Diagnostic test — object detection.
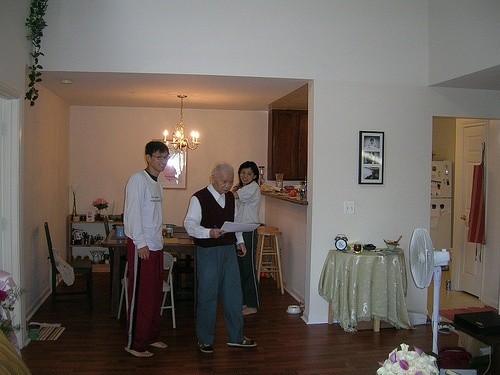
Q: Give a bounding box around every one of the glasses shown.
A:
[150,155,170,160]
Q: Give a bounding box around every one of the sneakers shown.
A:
[198,342,213,353]
[226,336,257,347]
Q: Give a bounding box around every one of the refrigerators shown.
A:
[430,161,451,270]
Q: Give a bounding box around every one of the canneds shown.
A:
[166,225,173,238]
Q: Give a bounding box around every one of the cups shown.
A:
[276,174,283,193]
[446,280,451,290]
[354,244,361,251]
[116,226,124,240]
[165,228,173,238]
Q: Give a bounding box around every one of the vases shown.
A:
[97,209,101,222]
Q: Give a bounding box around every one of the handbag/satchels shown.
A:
[52,248,74,286]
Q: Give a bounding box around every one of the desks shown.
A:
[102,227,197,316]
[318,248,416,332]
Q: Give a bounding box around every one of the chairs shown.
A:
[44,222,93,316]
[118,251,176,328]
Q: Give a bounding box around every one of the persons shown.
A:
[230,160,261,315]
[183,164,257,353]
[368,138,376,149]
[122,141,171,358]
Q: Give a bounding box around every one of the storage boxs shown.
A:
[453,311,500,349]
[458,330,491,358]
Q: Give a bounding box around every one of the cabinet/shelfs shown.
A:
[267,109,308,181]
[67,214,125,273]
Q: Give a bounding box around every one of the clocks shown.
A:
[335,233,349,252]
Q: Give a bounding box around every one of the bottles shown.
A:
[259,166,265,186]
[300,181,305,197]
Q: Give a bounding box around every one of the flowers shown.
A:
[376,343,440,375]
[91,197,109,210]
[0,288,26,338]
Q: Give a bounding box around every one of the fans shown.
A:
[409,227,450,355]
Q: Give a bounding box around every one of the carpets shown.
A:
[439,305,499,322]
[27,322,65,341]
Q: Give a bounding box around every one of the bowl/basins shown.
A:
[286,305,301,314]
[386,244,397,249]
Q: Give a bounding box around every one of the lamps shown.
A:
[162,94,200,151]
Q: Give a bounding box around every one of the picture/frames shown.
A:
[153,138,187,189]
[359,131,384,185]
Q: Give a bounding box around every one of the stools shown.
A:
[256,225,284,295]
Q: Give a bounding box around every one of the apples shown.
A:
[290,190,297,197]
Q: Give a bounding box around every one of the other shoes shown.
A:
[242,305,257,315]
[124,347,154,357]
[148,342,168,348]
